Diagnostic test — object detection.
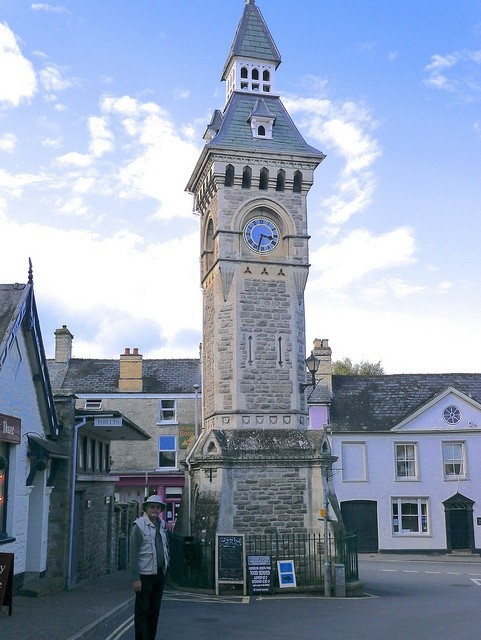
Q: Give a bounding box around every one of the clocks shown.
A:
[242,215,282,257]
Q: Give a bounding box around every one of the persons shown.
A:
[130,495,170,639]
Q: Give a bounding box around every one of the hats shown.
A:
[143,495,166,512]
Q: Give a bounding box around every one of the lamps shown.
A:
[302,350,322,391]
[22,431,47,471]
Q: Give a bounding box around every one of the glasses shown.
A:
[146,505,162,509]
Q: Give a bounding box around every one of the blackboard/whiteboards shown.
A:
[215,533,247,584]
[248,555,276,595]
